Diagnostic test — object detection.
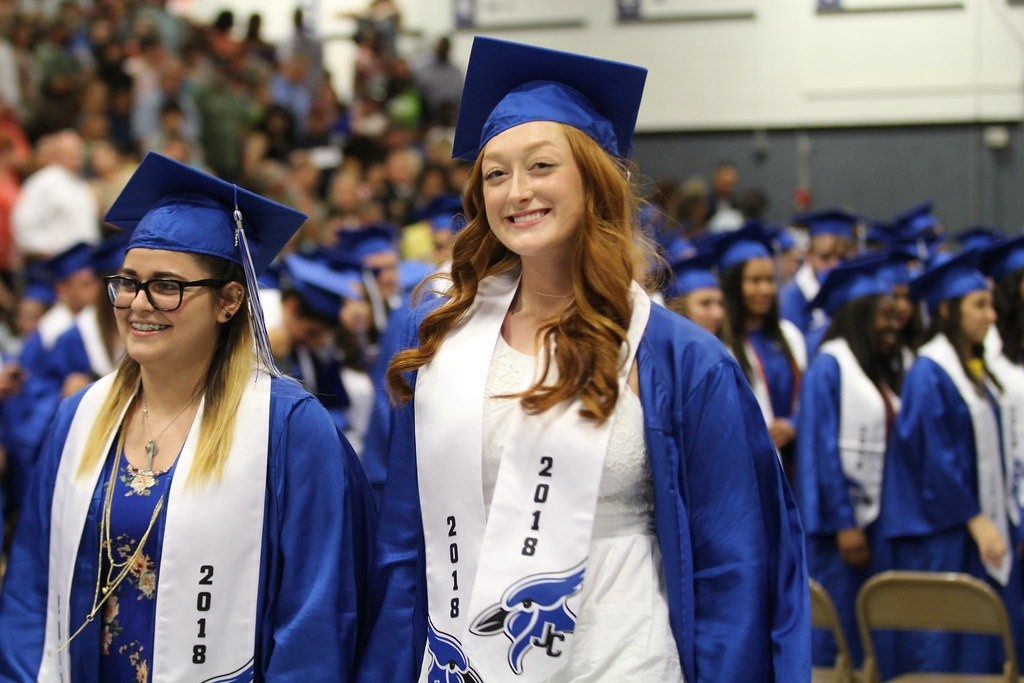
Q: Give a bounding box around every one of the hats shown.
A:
[104,151,309,383]
[649,219,793,301]
[797,198,1024,315]
[44,242,100,279]
[429,194,470,231]
[451,35,647,175]
[287,220,401,319]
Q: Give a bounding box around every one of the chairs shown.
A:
[807,575,854,683]
[853,569,1024,683]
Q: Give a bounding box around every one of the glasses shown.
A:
[105,274,220,312]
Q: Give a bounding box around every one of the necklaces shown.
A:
[139,392,203,471]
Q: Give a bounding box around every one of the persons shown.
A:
[0,152,356,683]
[795,258,915,672]
[886,247,1021,675]
[0,1,1024,505]
[350,36,811,683]
